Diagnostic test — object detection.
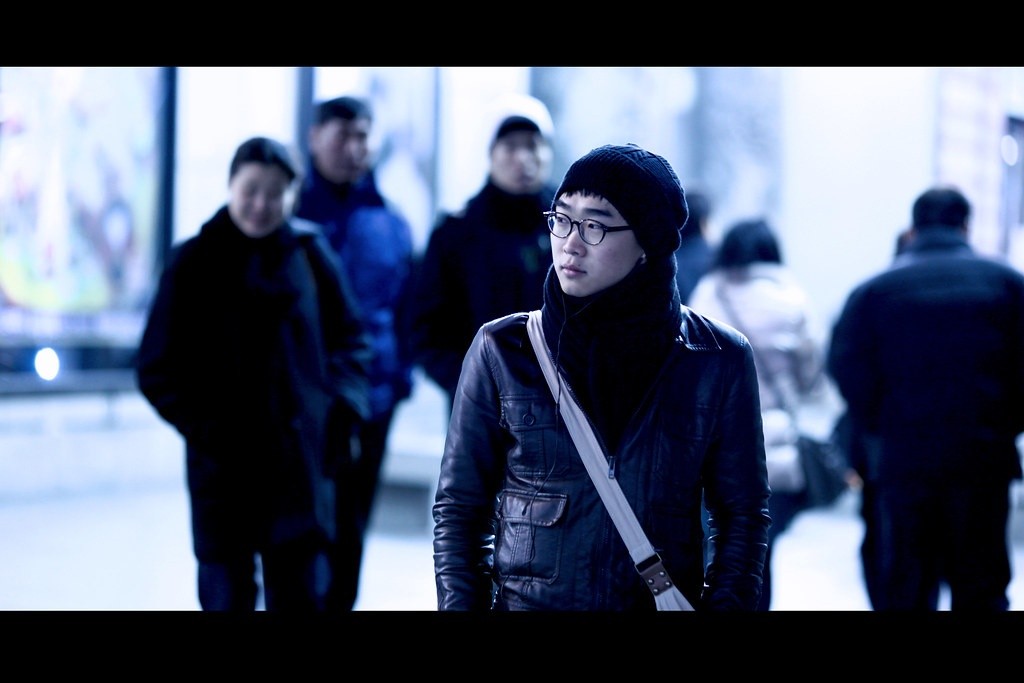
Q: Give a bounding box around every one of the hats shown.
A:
[550,142,689,269]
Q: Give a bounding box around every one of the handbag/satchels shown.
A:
[797,436,848,507]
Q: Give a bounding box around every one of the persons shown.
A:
[135,138,368,611]
[823,187,1023,613]
[432,144,771,612]
[676,194,717,305]
[290,98,415,611]
[685,221,848,541]
[397,96,563,419]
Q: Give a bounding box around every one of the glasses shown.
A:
[543,211,631,246]
